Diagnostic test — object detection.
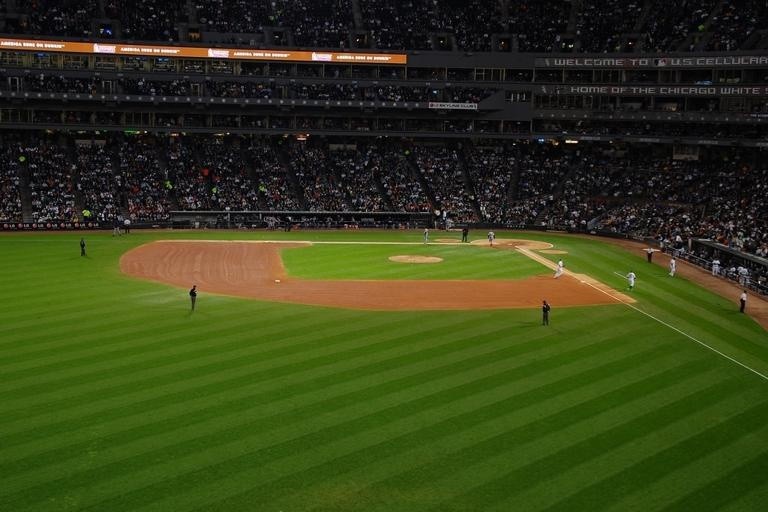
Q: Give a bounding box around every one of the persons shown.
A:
[541,299,551,326]
[423,226,429,246]
[461,226,468,242]
[538,1,767,302]
[284,214,293,232]
[3,0,536,230]
[189,285,198,311]
[739,289,748,313]
[79,237,86,256]
[112,217,122,236]
[124,217,131,235]
[487,229,495,247]
